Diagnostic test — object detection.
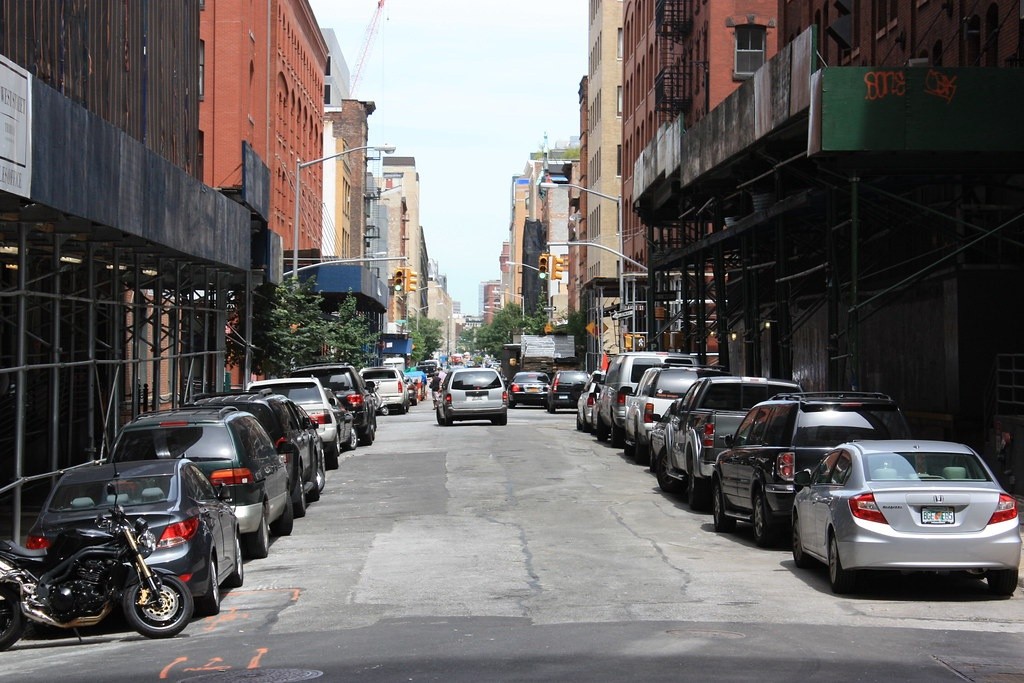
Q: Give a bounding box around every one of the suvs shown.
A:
[290,363,377,445]
[590,353,700,449]
[712,391,927,548]
[106,375,340,559]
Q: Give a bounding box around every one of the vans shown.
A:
[434,368,509,427]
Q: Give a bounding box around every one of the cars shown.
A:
[357,355,427,418]
[507,371,590,414]
[324,388,358,450]
[27,458,244,615]
[792,440,1022,596]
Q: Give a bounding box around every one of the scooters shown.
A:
[373,380,389,416]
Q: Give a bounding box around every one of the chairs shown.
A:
[141,487,165,502]
[875,468,899,479]
[106,494,128,504]
[70,498,95,509]
[942,466,966,480]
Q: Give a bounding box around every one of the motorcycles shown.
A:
[0,483,194,652]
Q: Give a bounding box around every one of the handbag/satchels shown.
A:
[429,383,433,388]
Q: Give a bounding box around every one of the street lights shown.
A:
[541,182,625,353]
[406,285,443,332]
[547,243,676,352]
[416,302,443,333]
[492,291,525,335]
[291,144,396,369]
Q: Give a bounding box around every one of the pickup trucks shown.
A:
[620,364,807,511]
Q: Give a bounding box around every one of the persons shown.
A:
[438,367,447,381]
[429,371,444,410]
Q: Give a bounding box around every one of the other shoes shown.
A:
[433,409,435,410]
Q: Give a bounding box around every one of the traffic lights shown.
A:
[404,267,417,294]
[393,269,404,296]
[551,255,564,280]
[539,255,548,280]
[623,333,645,349]
